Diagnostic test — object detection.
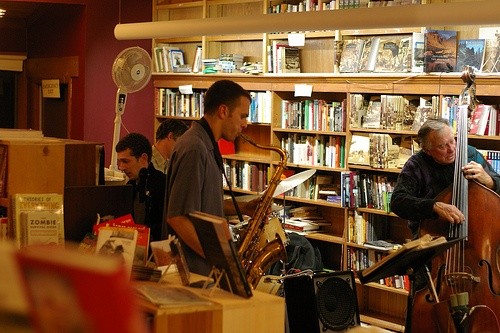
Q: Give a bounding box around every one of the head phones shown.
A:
[139,167,157,179]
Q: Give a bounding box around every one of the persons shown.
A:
[115,80,252,275]
[390,115,500,333]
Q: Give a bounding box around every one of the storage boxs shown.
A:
[13,239,138,333]
[130,282,224,333]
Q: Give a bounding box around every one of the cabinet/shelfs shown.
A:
[152,0,500,333]
[0,136,104,244]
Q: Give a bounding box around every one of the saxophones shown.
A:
[238,132,289,292]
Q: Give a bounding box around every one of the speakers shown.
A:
[282,270,360,333]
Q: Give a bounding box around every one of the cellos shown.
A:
[404,69,500,333]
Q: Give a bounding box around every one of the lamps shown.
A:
[112,0,500,40]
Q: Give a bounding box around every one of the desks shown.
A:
[162,271,286,333]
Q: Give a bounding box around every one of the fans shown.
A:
[103,45,153,182]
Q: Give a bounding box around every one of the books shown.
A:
[362,234,447,277]
[8,192,65,249]
[350,94,440,129]
[334,27,500,73]
[275,170,396,213]
[347,134,421,168]
[204,40,302,73]
[156,88,346,131]
[441,97,497,136]
[477,150,500,174]
[223,159,269,192]
[217,138,235,156]
[275,203,332,235]
[280,132,344,168]
[346,209,410,290]
[154,45,202,73]
[268,0,412,13]
[77,213,253,307]
